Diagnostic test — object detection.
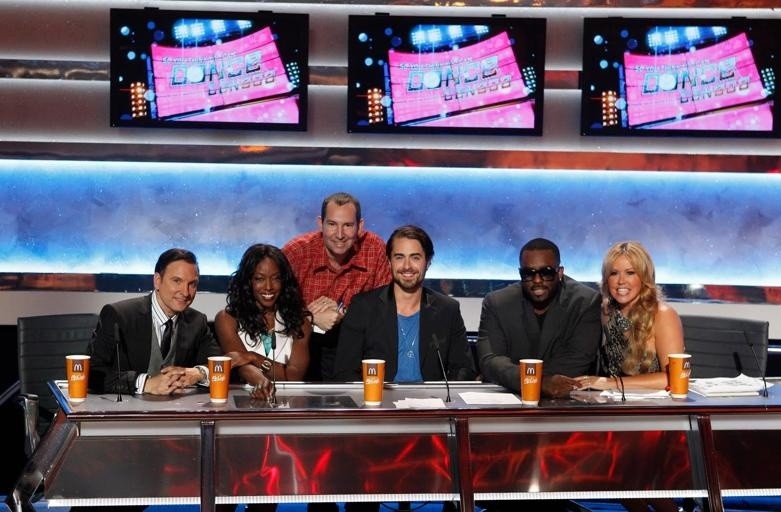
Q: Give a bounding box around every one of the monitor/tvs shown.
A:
[108,6,311,132]
[580,16,777,140]
[346,12,547,138]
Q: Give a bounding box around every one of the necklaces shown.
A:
[392,285,423,361]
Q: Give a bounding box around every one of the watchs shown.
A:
[195,367,206,383]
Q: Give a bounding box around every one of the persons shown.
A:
[477,237,602,397]
[573,241,683,391]
[214,244,312,401]
[280,192,393,333]
[334,226,476,382]
[84,248,223,396]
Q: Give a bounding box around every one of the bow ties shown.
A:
[161,320,173,360]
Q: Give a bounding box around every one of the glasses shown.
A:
[518,265,559,281]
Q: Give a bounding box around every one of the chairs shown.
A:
[679,313,769,381]
[16,312,99,432]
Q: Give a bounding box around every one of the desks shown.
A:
[49,375,781,511]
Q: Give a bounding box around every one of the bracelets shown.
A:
[260,357,273,374]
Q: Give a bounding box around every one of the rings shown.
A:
[323,302,328,305]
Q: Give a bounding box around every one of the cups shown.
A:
[668,353,692,400]
[208,356,231,403]
[362,359,385,406]
[519,359,543,406]
[65,355,91,402]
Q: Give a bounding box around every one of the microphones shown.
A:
[430,332,452,403]
[603,322,626,402]
[112,321,124,403]
[270,328,278,407]
[742,328,769,399]
